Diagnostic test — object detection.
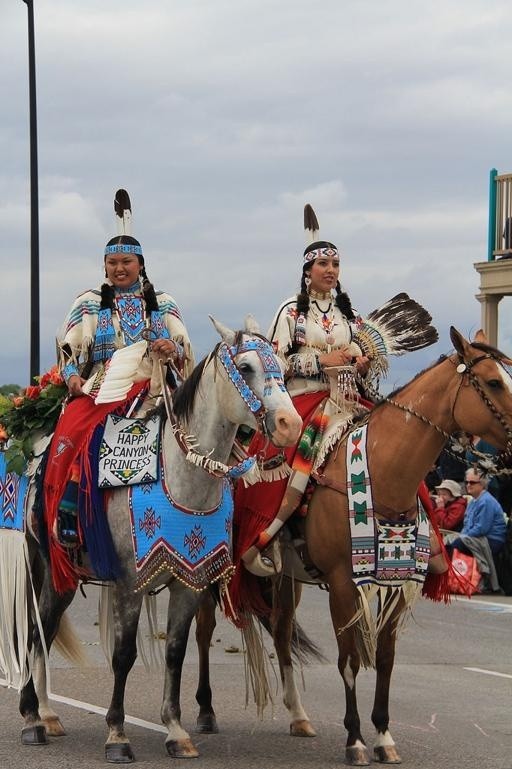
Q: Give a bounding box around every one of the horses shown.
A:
[194,323,512,768]
[0,314,305,769]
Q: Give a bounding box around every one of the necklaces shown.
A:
[309,289,337,324]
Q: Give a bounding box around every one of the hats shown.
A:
[434,478,464,498]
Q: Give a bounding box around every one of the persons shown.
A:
[424,431,511,594]
[35,235,195,551]
[242,241,449,576]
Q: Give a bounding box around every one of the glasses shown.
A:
[462,479,482,486]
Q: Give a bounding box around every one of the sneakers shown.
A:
[51,507,81,549]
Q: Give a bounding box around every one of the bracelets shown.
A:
[61,364,79,386]
[171,340,184,358]
[315,354,322,371]
[358,369,371,382]
